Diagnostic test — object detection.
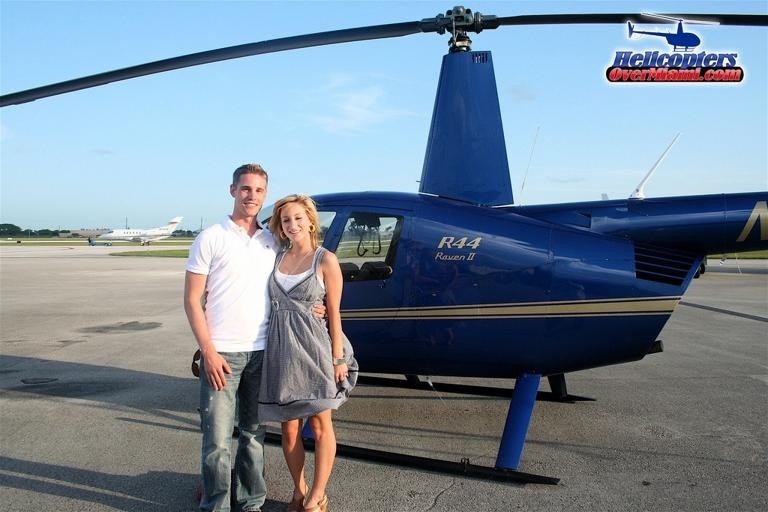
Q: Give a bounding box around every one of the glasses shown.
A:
[191,349,201,377]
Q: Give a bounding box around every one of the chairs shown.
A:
[338,261,393,282]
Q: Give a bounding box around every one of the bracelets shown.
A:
[332,358,347,365]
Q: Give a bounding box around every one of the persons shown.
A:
[257,192,361,512]
[182,161,330,512]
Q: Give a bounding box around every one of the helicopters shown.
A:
[0,6,768,471]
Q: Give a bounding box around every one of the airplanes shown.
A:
[99,215,185,246]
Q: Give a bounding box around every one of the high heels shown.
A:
[287,487,328,512]
